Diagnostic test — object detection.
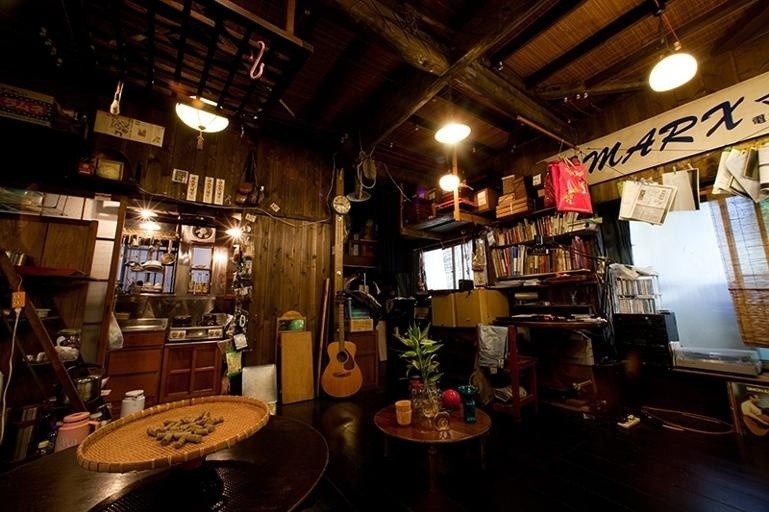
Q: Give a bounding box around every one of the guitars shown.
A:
[321,292,363,399]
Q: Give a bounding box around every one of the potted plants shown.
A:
[394,322,445,430]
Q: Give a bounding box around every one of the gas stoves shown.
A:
[168,324,224,343]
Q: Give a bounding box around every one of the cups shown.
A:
[396,400,411,426]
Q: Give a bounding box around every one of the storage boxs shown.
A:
[455,290,510,328]
[431,293,456,328]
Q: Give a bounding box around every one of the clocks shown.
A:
[332,195,351,215]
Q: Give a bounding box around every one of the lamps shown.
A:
[175,99,230,133]
[648,7,698,92]
[439,166,460,193]
[434,84,471,146]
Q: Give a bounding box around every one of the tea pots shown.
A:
[67,362,104,405]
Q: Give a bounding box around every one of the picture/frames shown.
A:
[95,159,125,180]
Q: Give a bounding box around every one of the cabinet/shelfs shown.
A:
[398,182,555,243]
[486,217,608,420]
[103,330,165,421]
[344,233,381,276]
[612,310,680,407]
[345,331,379,392]
[158,344,222,402]
[0,214,98,329]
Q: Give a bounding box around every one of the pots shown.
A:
[161,240,176,265]
[197,314,217,325]
[173,313,191,325]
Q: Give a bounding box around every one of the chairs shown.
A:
[477,324,538,424]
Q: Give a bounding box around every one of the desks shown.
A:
[672,365,769,434]
[375,403,492,512]
[1,415,330,512]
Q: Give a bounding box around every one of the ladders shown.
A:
[0,246,113,428]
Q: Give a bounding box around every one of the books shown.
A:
[491,238,593,278]
[495,211,597,246]
[616,276,660,314]
[496,197,533,218]
